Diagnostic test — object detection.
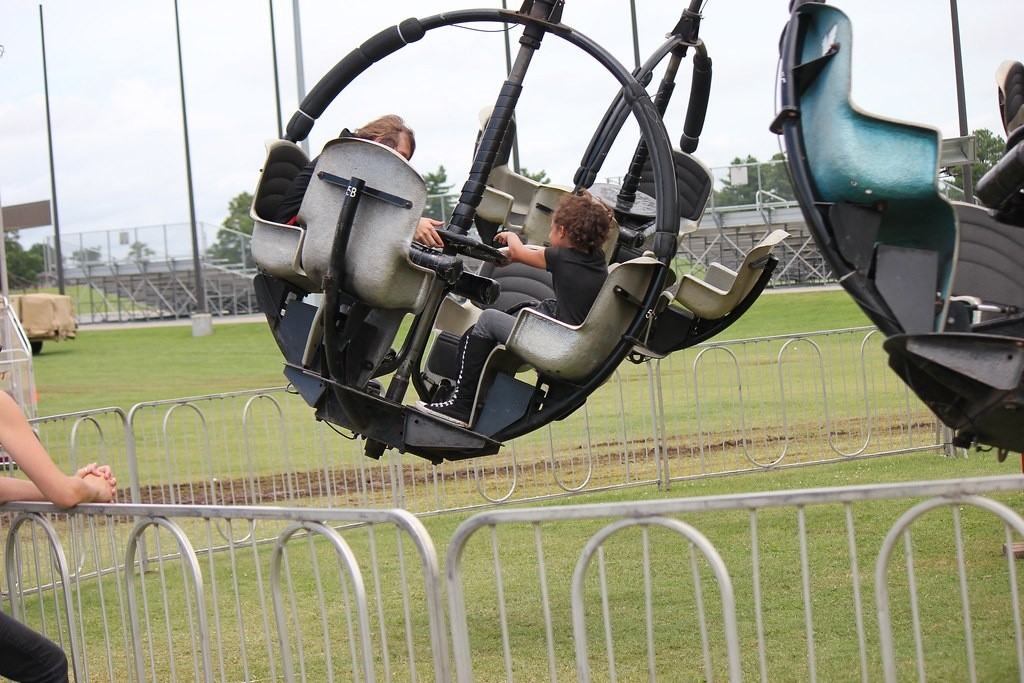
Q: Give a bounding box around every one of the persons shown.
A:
[0,344,118,683]
[413,187,615,428]
[274,113,446,396]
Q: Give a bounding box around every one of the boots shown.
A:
[415,334,499,426]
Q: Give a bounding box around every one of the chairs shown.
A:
[796,1,943,221]
[250,104,791,429]
